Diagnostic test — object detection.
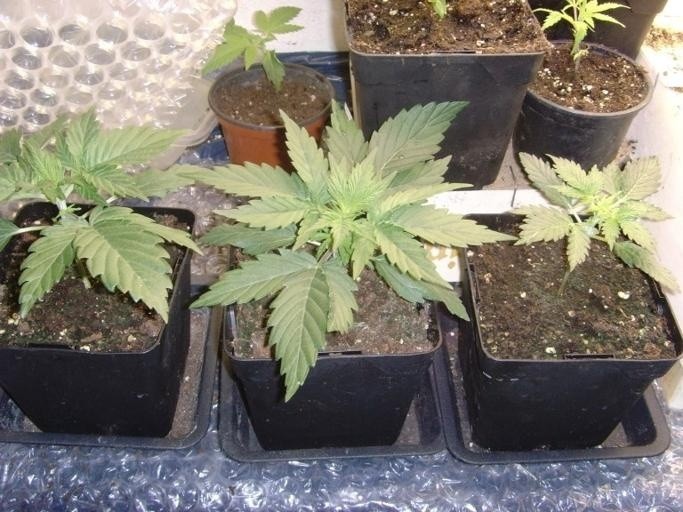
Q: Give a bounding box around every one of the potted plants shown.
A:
[336,0,549,186]
[206,8,342,168]
[195,97,508,455]
[0,110,212,436]
[523,0,675,173]
[462,149,683,452]
